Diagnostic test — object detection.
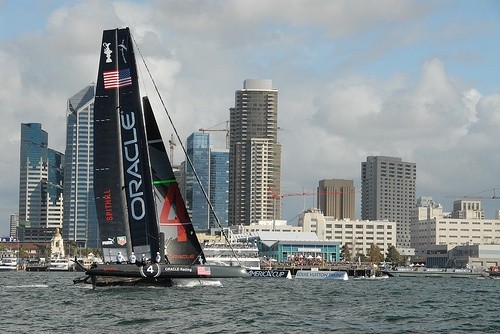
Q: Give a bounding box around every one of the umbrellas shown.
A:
[299,255,322,259]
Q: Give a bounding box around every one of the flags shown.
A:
[103,69,132,88]
[198,267,210,275]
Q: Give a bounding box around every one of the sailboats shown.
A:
[72,27,252,287]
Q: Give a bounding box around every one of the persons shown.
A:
[116,252,124,264]
[155,252,161,262]
[129,252,136,264]
[141,254,149,264]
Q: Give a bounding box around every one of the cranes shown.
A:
[198,120,229,134]
[169,133,177,165]
